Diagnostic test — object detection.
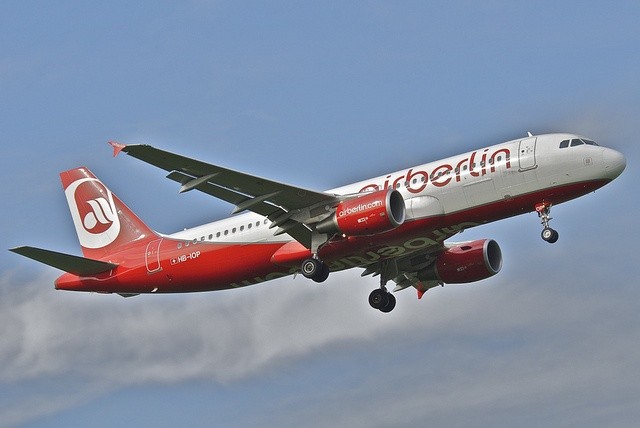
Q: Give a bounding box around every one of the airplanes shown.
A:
[8,130,627,313]
